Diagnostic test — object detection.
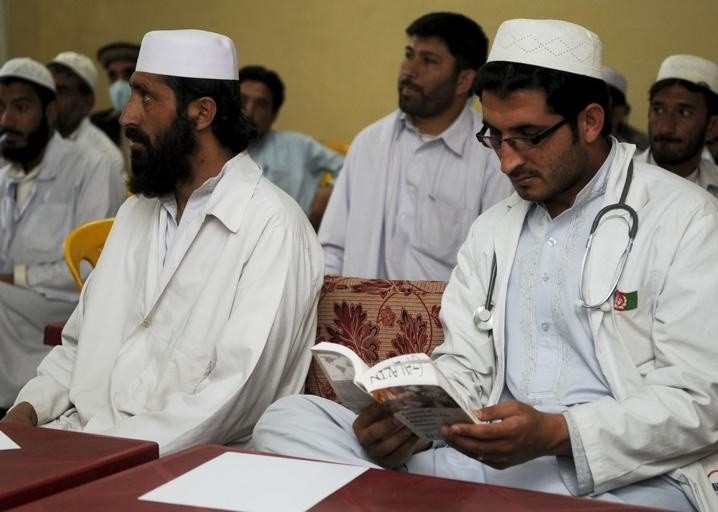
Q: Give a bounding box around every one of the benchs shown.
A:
[300,273,448,416]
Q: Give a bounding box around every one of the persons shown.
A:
[0,28,325,456]
[600,66,651,153]
[628,54,717,198]
[251,20,717,511]
[238,64,345,219]
[91,42,140,176]
[316,13,517,282]
[0,56,126,407]
[44,50,125,196]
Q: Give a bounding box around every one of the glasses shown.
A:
[476,117,570,154]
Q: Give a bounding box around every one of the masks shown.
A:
[108,79,132,114]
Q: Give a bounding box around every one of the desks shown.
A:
[4,444,676,512]
[1,420,159,512]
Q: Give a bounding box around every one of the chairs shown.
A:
[63,214,117,293]
[316,138,350,187]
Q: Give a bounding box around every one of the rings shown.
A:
[478,454,485,462]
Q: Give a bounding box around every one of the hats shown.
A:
[601,68,627,97]
[48,51,98,95]
[0,57,56,92]
[97,41,140,69]
[134,29,240,82]
[485,18,603,80]
[656,54,718,93]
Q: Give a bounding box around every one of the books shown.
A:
[311,340,481,445]
